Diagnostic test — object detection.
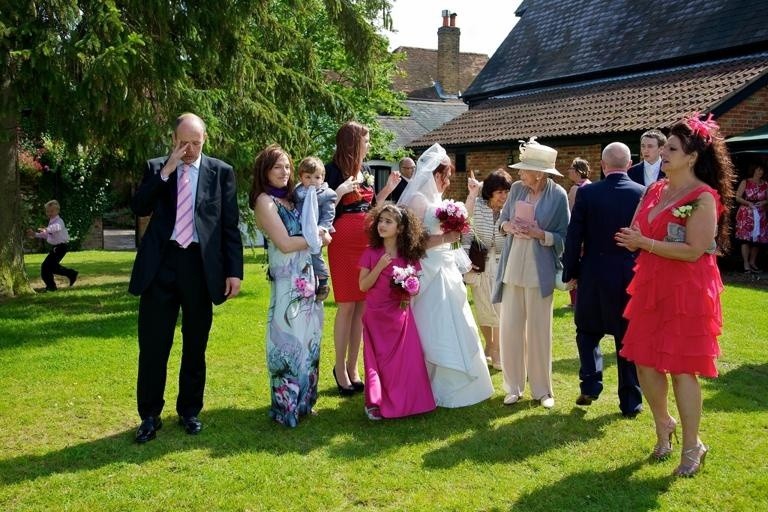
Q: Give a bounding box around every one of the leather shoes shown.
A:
[179,413,202,433]
[70,270,78,286]
[576,393,597,405]
[135,414,163,443]
[504,393,518,404]
[540,394,554,408]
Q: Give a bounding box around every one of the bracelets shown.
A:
[441,234,446,244]
[649,239,656,254]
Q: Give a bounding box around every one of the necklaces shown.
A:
[527,191,543,204]
[664,180,693,207]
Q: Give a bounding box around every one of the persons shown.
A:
[562,156,592,308]
[358,201,437,420]
[627,129,667,186]
[129,113,243,443]
[26,199,79,292]
[291,156,337,303]
[615,112,736,476]
[560,142,649,418]
[324,122,401,395]
[490,136,571,409]
[248,142,332,429]
[735,163,768,273]
[472,168,511,370]
[395,142,496,408]
[385,157,416,206]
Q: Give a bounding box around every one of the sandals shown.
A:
[364,406,381,421]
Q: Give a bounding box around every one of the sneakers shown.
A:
[317,283,330,300]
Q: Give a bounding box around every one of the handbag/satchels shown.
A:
[461,244,481,287]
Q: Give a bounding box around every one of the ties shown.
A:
[175,163,194,248]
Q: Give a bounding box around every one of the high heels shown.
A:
[484,342,492,366]
[653,417,678,460]
[346,366,364,393]
[749,263,762,273]
[744,269,752,275]
[673,439,709,477]
[492,350,503,371]
[333,366,355,395]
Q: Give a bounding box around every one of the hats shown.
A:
[508,135,565,178]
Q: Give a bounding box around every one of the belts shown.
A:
[345,205,369,212]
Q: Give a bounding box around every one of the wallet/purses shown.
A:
[513,201,535,239]
